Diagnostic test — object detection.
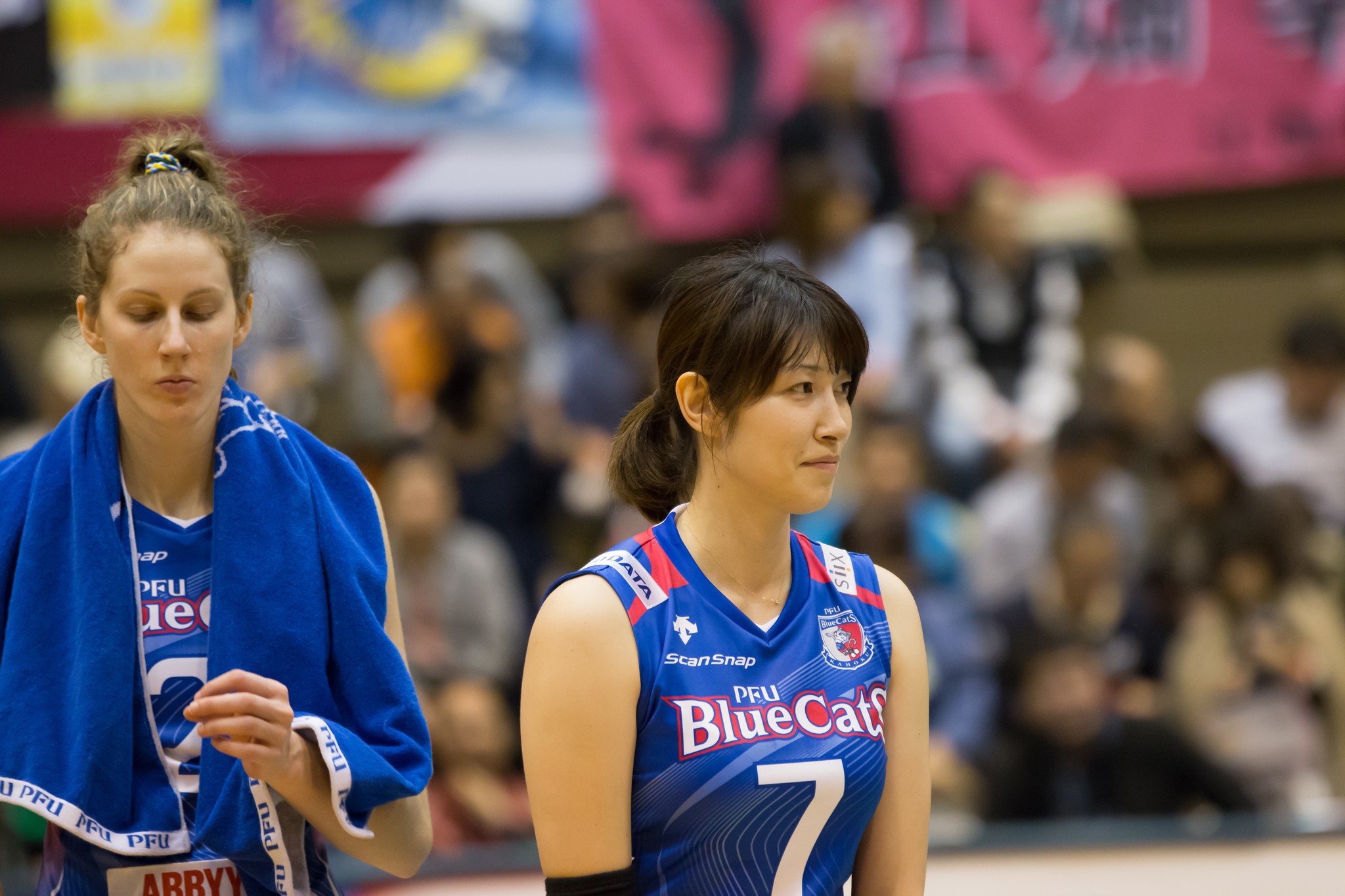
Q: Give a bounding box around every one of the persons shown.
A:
[0,126,435,896]
[519,250,930,896]
[0,27,1345,850]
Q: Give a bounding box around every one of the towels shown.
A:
[0,374,436,896]
